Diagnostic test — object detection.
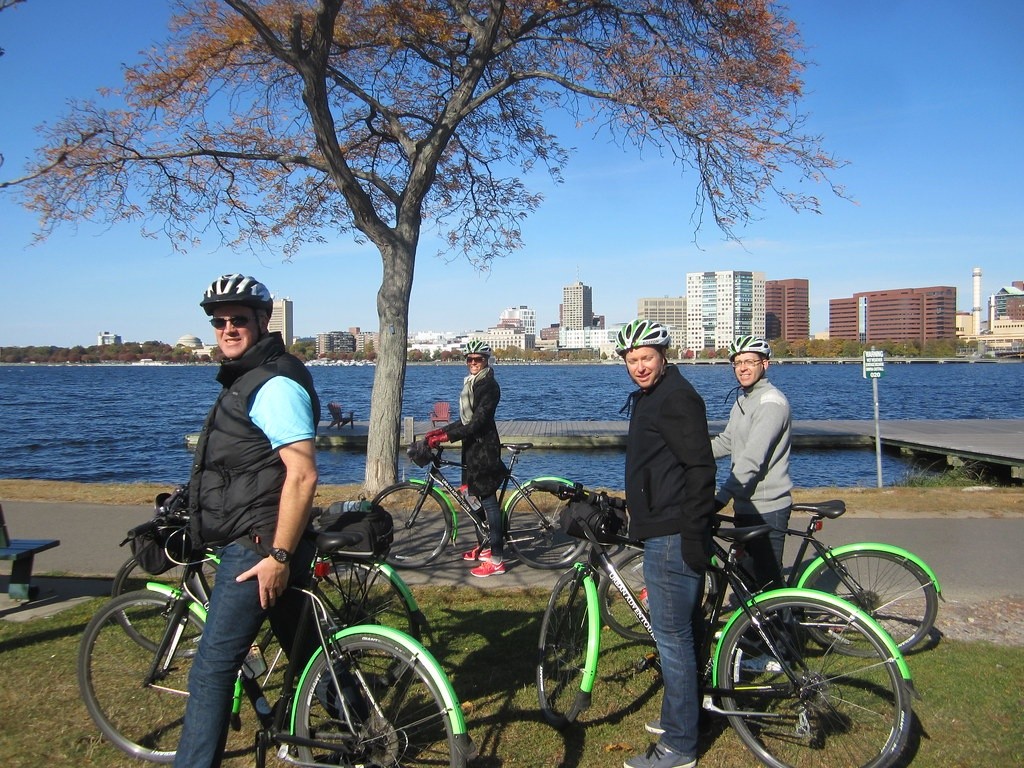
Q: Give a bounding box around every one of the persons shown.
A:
[608,321,718,768]
[711,333,795,675]
[424,340,509,578]
[172,271,321,768]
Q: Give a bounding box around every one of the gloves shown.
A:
[425,428,443,439]
[681,536,711,573]
[428,432,449,448]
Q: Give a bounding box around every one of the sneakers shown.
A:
[624,740,696,768]
[645,720,666,734]
[469,559,506,577]
[741,645,792,674]
[462,545,492,562]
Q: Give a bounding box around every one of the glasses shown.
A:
[467,357,483,363]
[208,316,256,330]
[732,359,763,367]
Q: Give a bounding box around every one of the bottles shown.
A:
[735,551,757,582]
[328,500,375,515]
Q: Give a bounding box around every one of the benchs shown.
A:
[0,501,61,602]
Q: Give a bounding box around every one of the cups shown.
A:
[239,639,269,680]
[460,484,481,511]
[639,587,651,615]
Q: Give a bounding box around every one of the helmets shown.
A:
[199,273,273,319]
[463,340,491,358]
[728,335,772,363]
[614,318,670,356]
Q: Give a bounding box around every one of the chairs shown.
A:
[430,401,453,428]
[327,402,355,430]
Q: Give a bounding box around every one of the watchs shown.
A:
[270,547,295,563]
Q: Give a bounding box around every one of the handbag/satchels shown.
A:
[312,495,394,555]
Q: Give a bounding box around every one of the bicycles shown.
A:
[525,479,921,768]
[598,500,945,659]
[372,439,590,572]
[77,477,477,768]
